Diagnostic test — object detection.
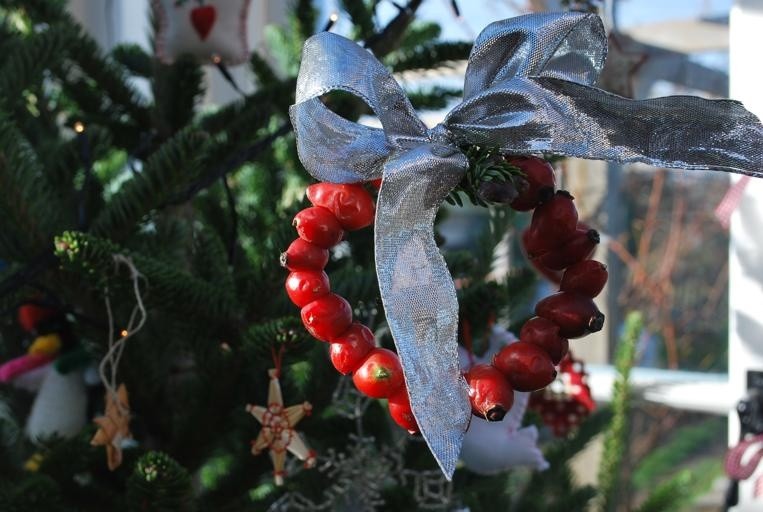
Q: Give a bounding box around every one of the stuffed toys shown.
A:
[1,302,127,483]
